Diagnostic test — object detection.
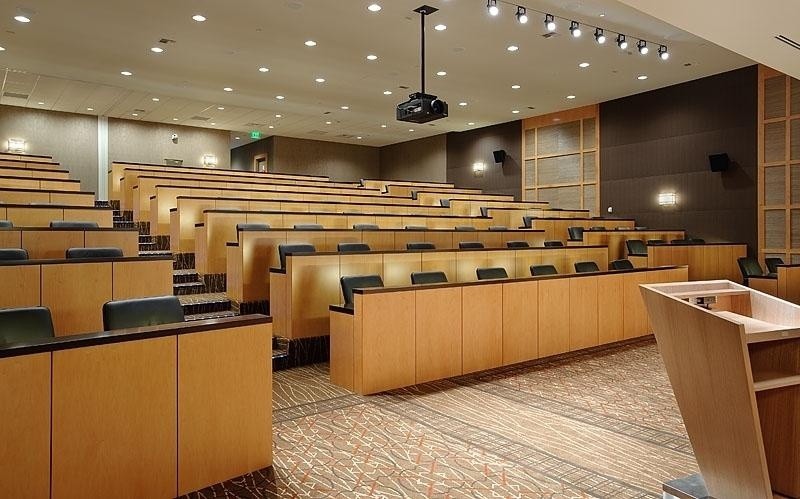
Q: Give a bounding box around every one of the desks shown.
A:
[2,250,175,339]
[748,262,798,306]
[270,245,609,338]
[3,312,273,498]
[1,154,747,278]
[327,265,690,396]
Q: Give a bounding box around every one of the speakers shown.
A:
[493,151,506,163]
[708,153,729,172]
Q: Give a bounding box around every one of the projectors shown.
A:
[396,98,449,124]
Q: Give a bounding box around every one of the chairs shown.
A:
[103,294,187,333]
[476,268,509,280]
[738,256,778,285]
[763,256,786,274]
[341,275,385,305]
[411,271,448,285]
[1,305,55,344]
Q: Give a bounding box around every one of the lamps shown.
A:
[250,130,261,139]
[487,0,670,61]
[7,139,25,152]
